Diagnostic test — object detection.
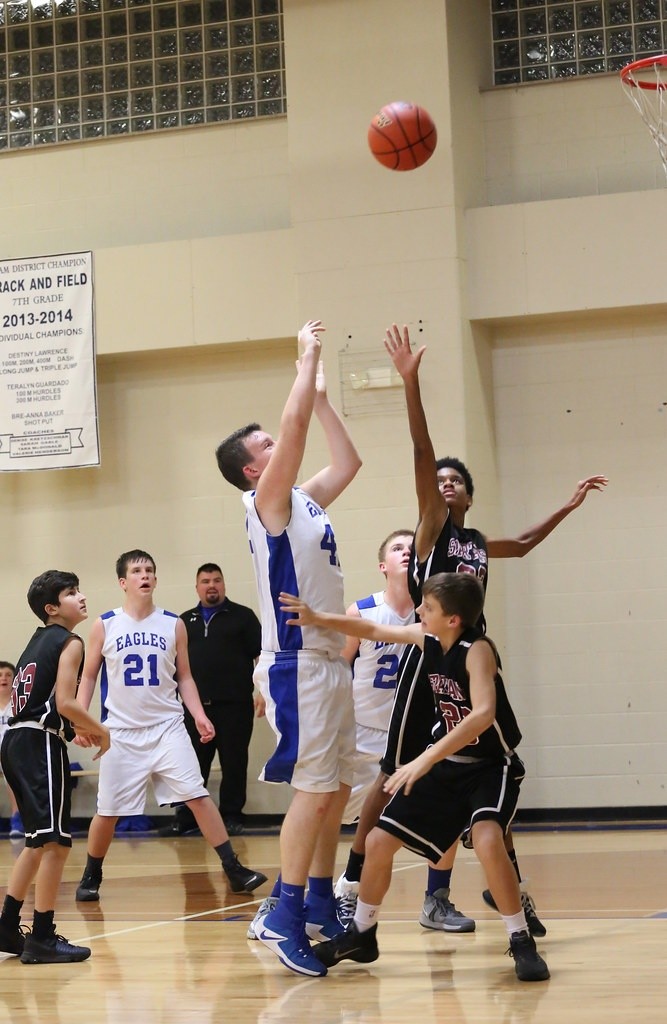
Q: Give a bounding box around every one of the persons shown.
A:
[0,661,24,836]
[158,563,262,834]
[1,569,109,965]
[333,325,609,933]
[216,321,362,977]
[334,529,476,933]
[277,572,551,983]
[75,549,267,902]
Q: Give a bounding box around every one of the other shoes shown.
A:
[170,823,199,835]
[223,817,245,834]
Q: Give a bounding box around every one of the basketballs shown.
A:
[368,101,438,171]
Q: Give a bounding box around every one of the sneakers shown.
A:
[334,890,360,929]
[10,838,25,853]
[22,933,90,962]
[253,913,327,977]
[505,931,550,981]
[258,978,322,1024]
[247,899,276,939]
[302,890,344,942]
[9,812,26,836]
[312,919,380,967]
[76,866,102,899]
[0,922,32,955]
[484,885,546,938]
[420,887,475,933]
[222,853,268,894]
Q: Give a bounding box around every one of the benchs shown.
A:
[0,766,222,783]
